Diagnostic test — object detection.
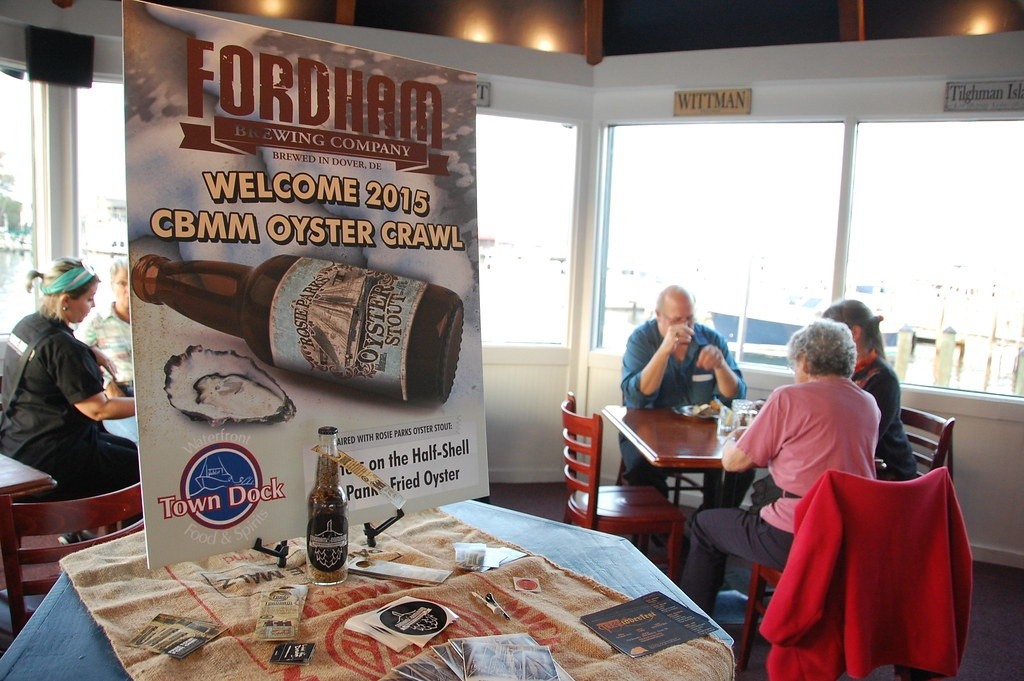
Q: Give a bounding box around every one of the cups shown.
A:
[736,410,757,427]
[729,397,753,428]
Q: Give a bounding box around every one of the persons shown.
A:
[819,297,920,481]
[0,256,142,544]
[679,316,883,618]
[618,283,759,564]
[75,258,135,396]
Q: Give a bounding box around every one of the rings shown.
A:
[675,330,680,336]
[675,338,679,341]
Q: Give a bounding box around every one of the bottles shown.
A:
[307,426,351,588]
[131,250,465,404]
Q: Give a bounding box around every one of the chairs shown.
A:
[898,405,956,481]
[560,391,687,586]
[1,478,147,642]
[737,468,952,672]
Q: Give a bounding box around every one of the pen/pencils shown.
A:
[485,592,510,620]
[471,591,500,615]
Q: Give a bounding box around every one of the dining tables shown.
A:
[0,496,737,681]
[600,403,754,570]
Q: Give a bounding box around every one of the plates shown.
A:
[670,403,721,419]
